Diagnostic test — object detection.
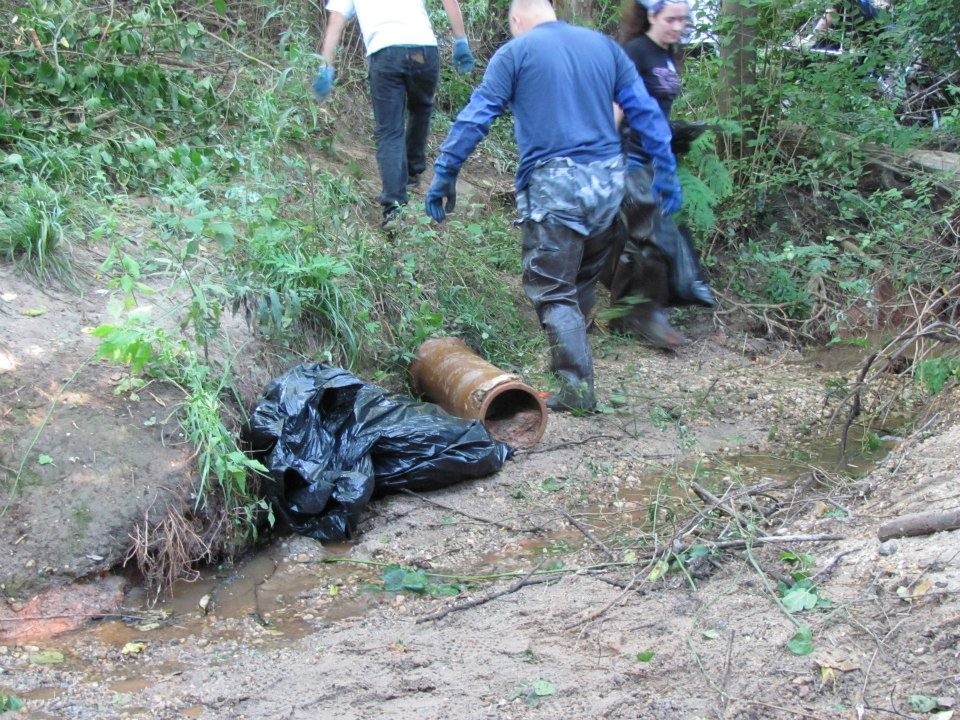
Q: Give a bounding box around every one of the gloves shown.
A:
[426,90,506,223]
[313,65,335,97]
[614,75,682,215]
[452,35,474,77]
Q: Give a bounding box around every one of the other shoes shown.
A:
[381,207,398,232]
[406,174,419,191]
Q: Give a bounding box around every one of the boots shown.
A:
[520,219,598,413]
[609,170,691,348]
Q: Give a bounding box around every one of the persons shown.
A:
[311,0,475,227]
[613,0,903,306]
[425,0,683,416]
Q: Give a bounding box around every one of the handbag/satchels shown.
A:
[655,216,716,309]
